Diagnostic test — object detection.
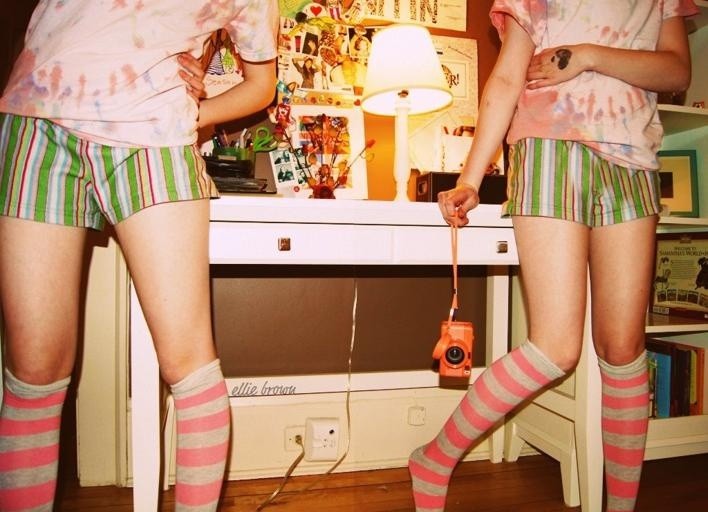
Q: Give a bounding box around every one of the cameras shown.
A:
[432,322,474,378]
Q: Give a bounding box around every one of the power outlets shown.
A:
[285,418,344,462]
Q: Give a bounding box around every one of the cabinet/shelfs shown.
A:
[504,0,708,507]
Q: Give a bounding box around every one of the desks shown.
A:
[132,194,605,512]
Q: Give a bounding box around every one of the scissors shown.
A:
[251,127,278,153]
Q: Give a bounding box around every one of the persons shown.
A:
[408,1,698,510]
[291,21,371,93]
[0,1,278,512]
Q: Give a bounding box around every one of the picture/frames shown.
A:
[656,149,699,216]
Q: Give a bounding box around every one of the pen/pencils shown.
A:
[212,127,253,152]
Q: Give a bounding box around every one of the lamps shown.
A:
[362,23,454,201]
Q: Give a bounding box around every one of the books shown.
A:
[640,237,708,419]
[206,156,270,193]
